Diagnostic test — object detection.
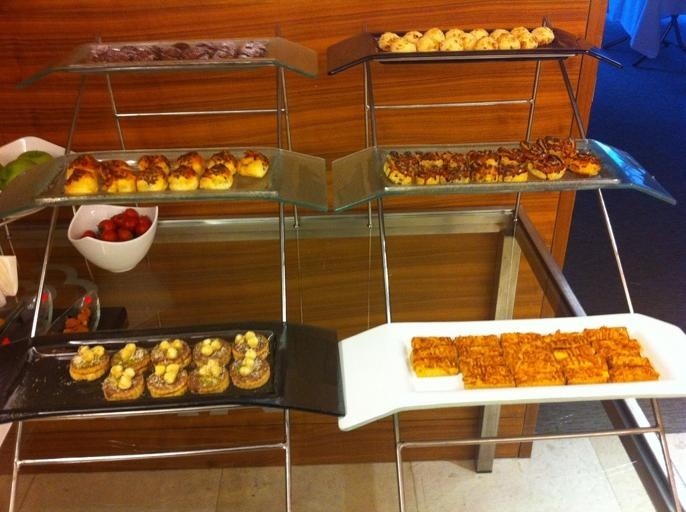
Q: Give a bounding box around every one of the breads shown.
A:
[408,325,659,389]
[97,40,266,63]
[69,328,272,402]
[377,26,555,53]
[384,135,600,185]
[63,149,269,196]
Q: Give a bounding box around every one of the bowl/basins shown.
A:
[66,204,159,274]
[0,134,76,218]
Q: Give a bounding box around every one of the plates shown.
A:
[337,312,686,432]
[15,36,319,87]
[326,26,623,77]
[0,146,327,222]
[331,139,680,214]
[0,321,345,425]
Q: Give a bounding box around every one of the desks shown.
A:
[602,0,686,67]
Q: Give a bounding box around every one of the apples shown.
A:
[0,151,51,196]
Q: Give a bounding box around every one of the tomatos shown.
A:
[80,209,151,239]
